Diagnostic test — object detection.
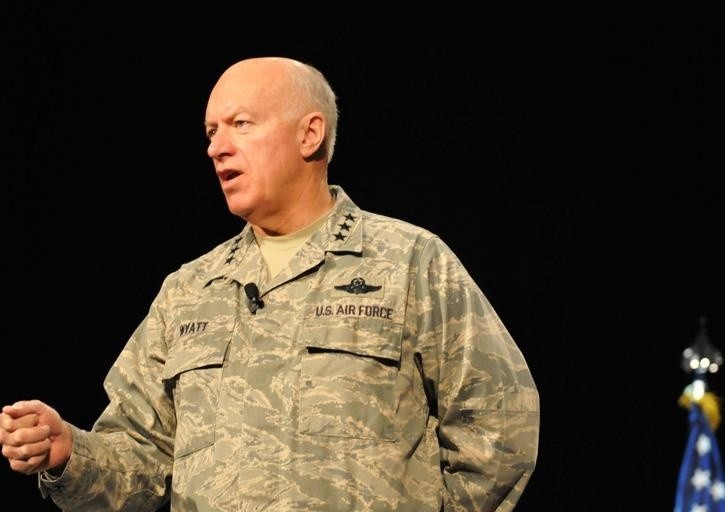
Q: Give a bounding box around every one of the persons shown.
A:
[1,55,540,512]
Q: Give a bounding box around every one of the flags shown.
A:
[674,405,724,511]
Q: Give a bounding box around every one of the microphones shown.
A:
[244,283,264,309]
